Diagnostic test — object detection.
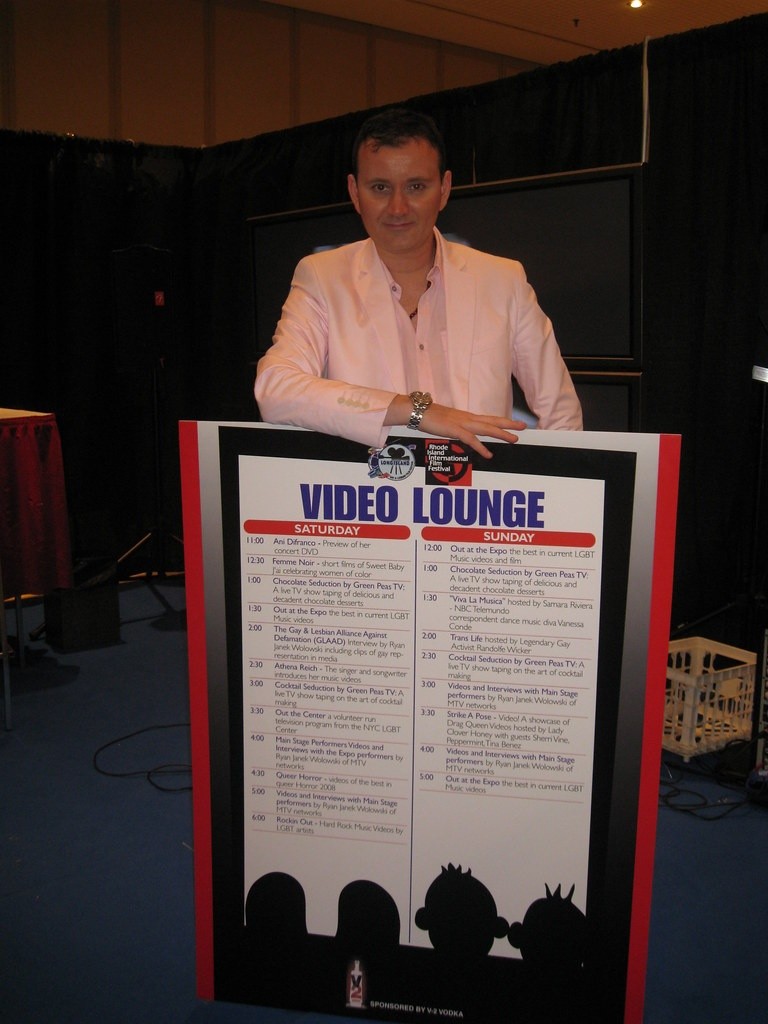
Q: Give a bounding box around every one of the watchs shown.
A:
[406,391,433,430]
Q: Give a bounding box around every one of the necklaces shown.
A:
[409,281,432,319]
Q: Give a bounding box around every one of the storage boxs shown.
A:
[661,635,758,764]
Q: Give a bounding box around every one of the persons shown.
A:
[254,109,584,460]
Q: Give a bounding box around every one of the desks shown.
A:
[1,408,73,733]
